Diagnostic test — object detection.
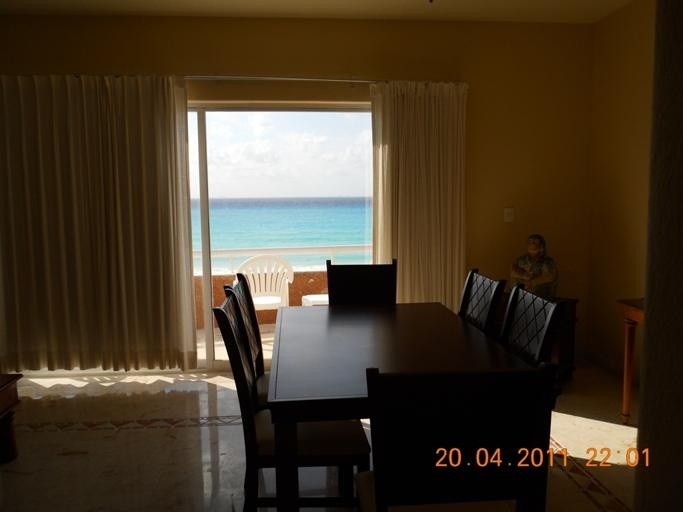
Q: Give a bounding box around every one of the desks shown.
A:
[615,299,645,427]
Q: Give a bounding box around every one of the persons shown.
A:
[508,232,557,298]
